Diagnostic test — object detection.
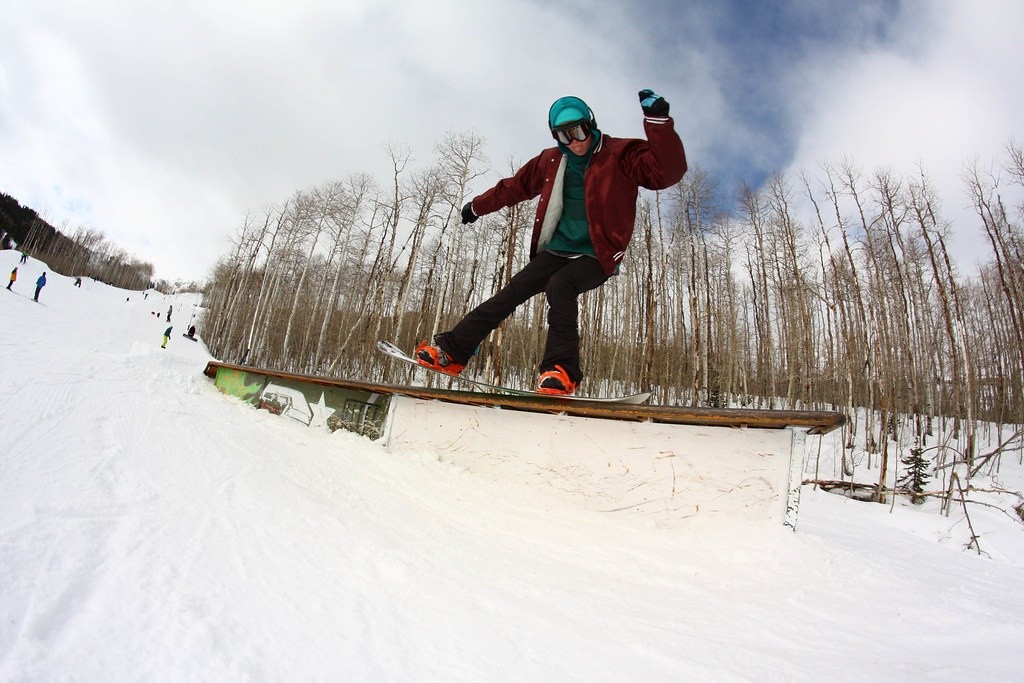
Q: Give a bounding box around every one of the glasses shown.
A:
[551,125,591,146]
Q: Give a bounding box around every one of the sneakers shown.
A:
[417,344,465,375]
[538,370,574,390]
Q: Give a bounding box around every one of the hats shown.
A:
[553,107,584,126]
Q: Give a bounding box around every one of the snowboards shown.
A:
[183,333,198,342]
[29,297,47,306]
[374,337,651,405]
[1,285,20,295]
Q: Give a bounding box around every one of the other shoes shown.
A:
[166,320,170,322]
[161,345,166,349]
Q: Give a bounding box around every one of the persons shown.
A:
[20,250,29,264]
[188,326,195,337]
[74,278,81,288]
[6,267,18,291]
[31,272,46,302]
[161,326,173,349]
[166,305,172,322]
[415,89,688,396]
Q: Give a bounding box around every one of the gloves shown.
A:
[460,201,480,225]
[638,89,671,119]
